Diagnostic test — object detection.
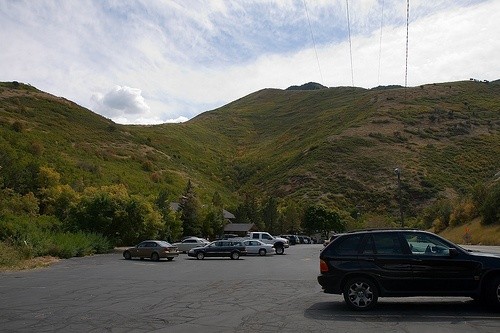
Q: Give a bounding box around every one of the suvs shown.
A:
[188,238,247,259]
[316,226,500,312]
[227,231,292,254]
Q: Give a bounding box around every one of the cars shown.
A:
[235,239,275,256]
[123,240,179,262]
[276,233,316,244]
[173,236,210,254]
